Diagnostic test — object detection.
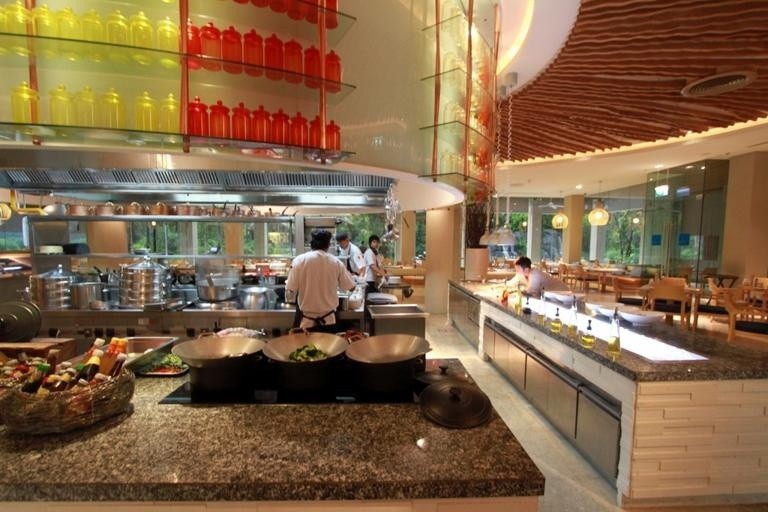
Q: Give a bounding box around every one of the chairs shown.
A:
[489,254,768,344]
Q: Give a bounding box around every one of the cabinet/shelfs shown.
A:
[0,1,357,165]
[25,209,305,303]
[444,281,619,487]
[416,1,499,206]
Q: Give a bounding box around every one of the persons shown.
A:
[284,228,355,333]
[364,234,386,293]
[334,231,367,280]
[359,239,366,253]
[506,256,569,297]
[378,225,398,254]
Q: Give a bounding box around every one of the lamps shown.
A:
[475,72,523,247]
[551,188,569,230]
[586,179,610,228]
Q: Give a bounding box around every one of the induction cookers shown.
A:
[158,368,415,405]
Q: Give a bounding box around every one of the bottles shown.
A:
[20,336,128,395]
[2,86,340,154]
[1,3,342,96]
[501,280,623,360]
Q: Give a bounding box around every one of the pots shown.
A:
[172,326,432,378]
[42,199,300,219]
[25,255,277,312]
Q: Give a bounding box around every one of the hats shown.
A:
[516,255,532,266]
[335,232,349,241]
[368,235,380,243]
[311,230,332,241]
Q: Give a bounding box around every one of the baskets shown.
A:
[3,360,135,436]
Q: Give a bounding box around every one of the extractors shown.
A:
[0,166,393,205]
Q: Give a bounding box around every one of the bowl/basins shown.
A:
[545,288,667,327]
